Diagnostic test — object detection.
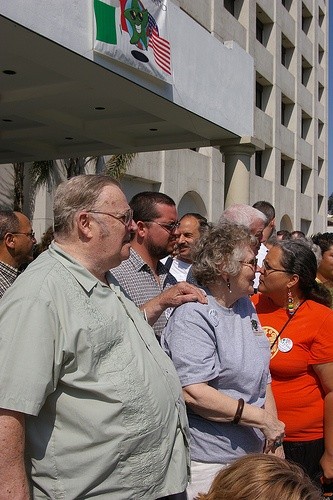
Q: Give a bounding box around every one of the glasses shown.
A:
[85,209,134,222]
[142,221,180,230]
[13,232,35,237]
[263,259,296,274]
[233,259,258,266]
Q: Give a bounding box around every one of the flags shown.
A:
[92,0,172,85]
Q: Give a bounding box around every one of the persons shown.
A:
[0,191,333,500]
[0,173,208,500]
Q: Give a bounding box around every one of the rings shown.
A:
[276,441,281,447]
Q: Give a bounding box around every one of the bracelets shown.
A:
[232,398,244,425]
